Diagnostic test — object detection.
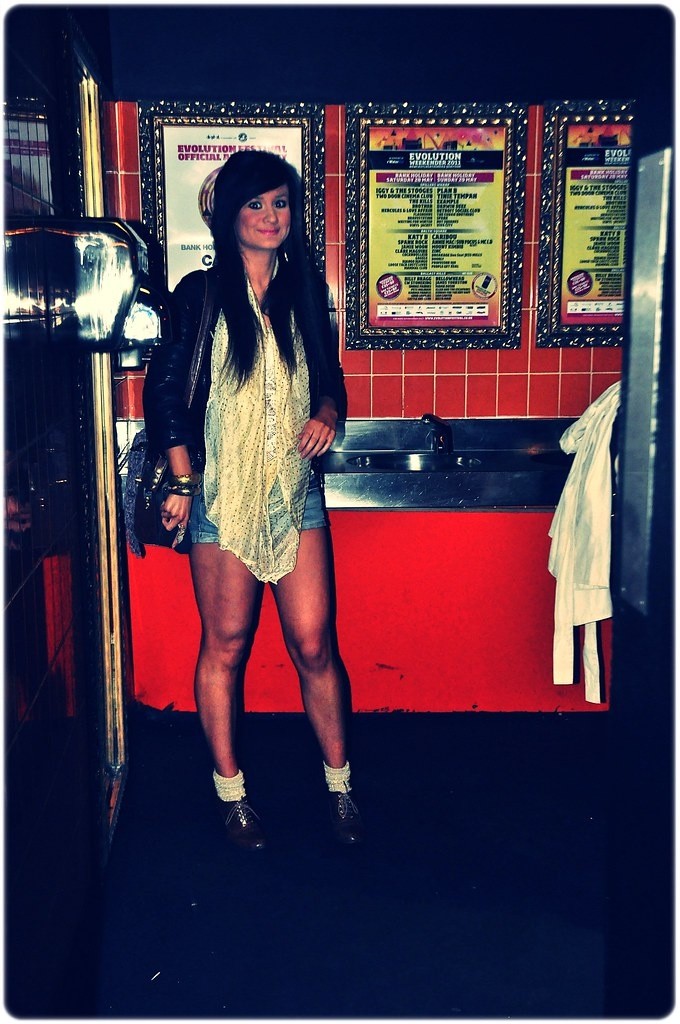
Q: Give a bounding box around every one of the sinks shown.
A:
[345,451,482,472]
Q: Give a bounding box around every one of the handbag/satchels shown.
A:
[121,428,192,554]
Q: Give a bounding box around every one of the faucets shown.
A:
[420,414,454,453]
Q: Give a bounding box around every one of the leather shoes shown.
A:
[209,787,265,852]
[320,783,363,844]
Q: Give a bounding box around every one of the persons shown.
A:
[142,148,365,855]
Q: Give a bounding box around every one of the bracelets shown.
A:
[167,473,198,496]
